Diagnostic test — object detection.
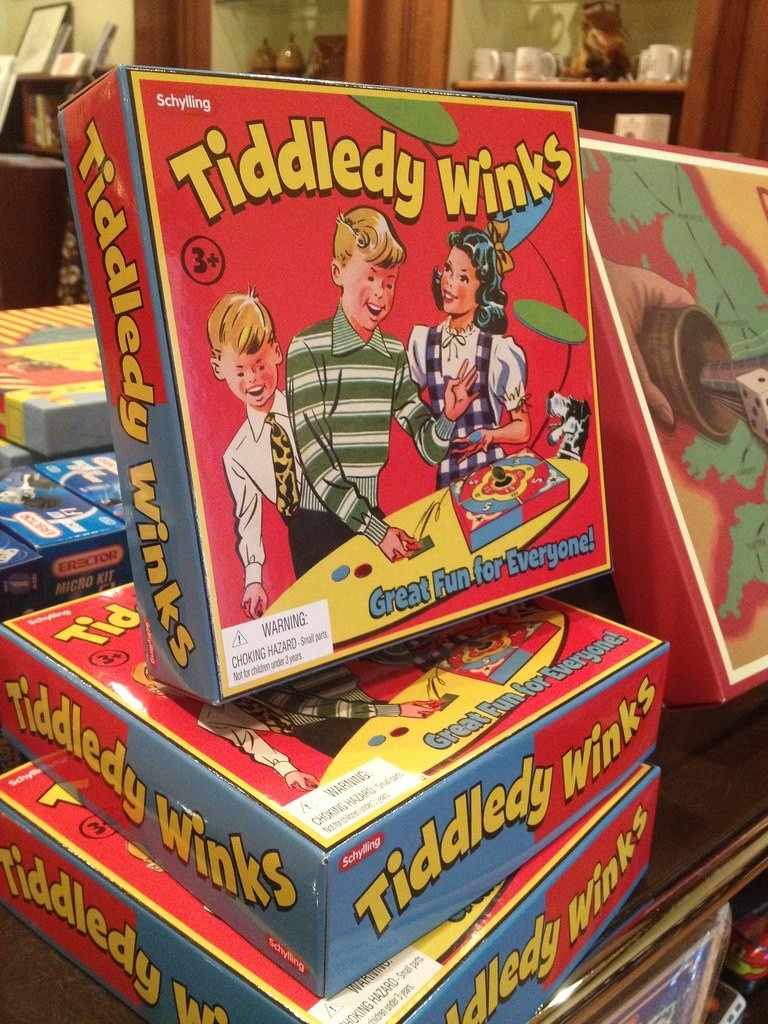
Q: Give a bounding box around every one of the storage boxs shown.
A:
[0,62,768,1024]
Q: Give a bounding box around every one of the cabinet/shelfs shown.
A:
[134,0,768,158]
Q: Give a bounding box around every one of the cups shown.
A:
[678,48,691,83]
[628,49,648,82]
[500,52,514,80]
[514,46,557,80]
[471,47,500,81]
[645,45,683,82]
